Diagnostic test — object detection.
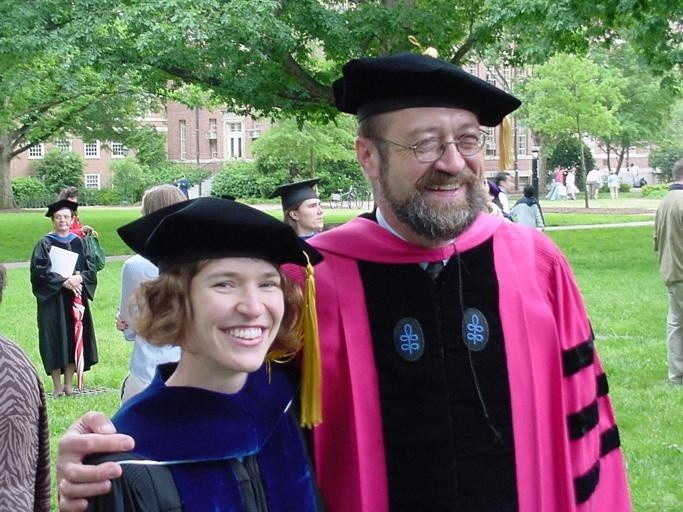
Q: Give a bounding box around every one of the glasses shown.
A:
[371,129,490,164]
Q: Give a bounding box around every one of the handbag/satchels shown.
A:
[85,237,105,271]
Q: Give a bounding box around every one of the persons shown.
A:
[484,163,641,231]
[178,175,191,200]
[30,199,99,398]
[116,184,187,407]
[57,53,632,512]
[0,264,51,512]
[652,159,683,385]
[81,196,330,512]
[59,187,98,239]
[269,178,323,240]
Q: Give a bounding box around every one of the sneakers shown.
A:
[53,388,77,401]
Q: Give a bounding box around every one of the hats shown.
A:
[117,195,325,274]
[331,53,522,127]
[268,178,323,211]
[45,200,78,217]
